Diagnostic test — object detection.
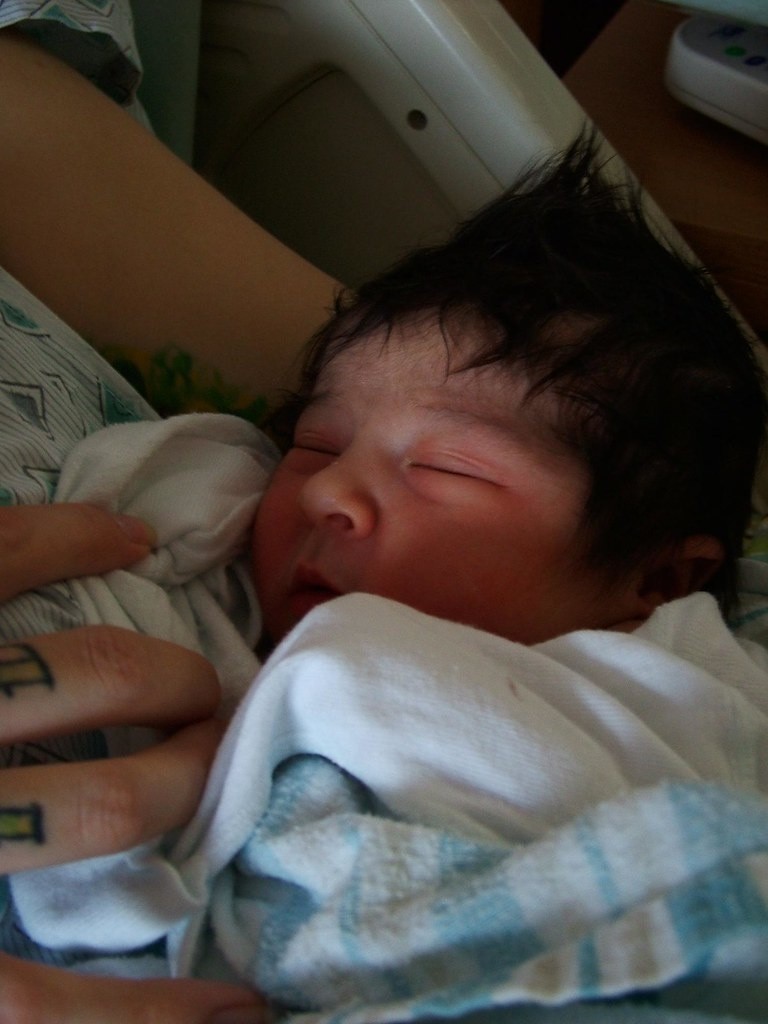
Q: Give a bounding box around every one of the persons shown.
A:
[0,0,768,1024]
